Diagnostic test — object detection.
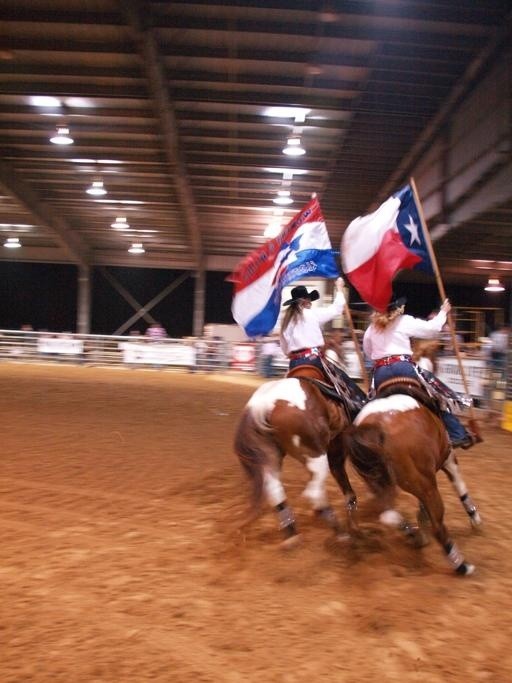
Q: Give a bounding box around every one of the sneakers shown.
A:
[459,432,481,449]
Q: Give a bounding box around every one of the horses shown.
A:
[339,333,486,578]
[208,330,369,552]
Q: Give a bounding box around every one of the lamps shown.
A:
[49,125,75,145]
[110,217,130,230]
[3,238,23,248]
[85,176,108,196]
[128,243,146,254]
[483,278,506,293]
[283,138,306,156]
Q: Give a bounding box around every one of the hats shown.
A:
[283,286,319,306]
[388,291,408,311]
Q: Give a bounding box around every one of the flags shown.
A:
[225,195,343,339]
[339,184,434,312]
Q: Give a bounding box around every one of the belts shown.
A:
[289,346,321,361]
[375,356,410,366]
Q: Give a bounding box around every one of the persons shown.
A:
[363,294,484,450]
[279,278,369,405]
[128,322,221,371]
[488,326,510,380]
[427,312,454,356]
